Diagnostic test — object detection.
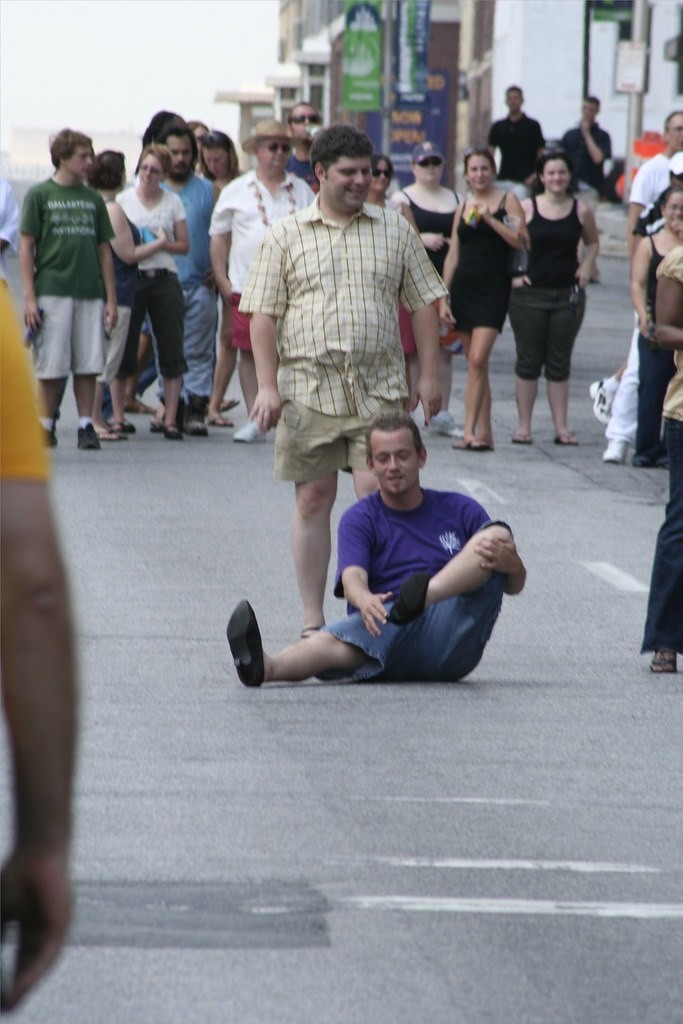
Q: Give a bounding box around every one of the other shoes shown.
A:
[162,422,183,440]
[232,419,267,443]
[424,411,466,438]
[41,423,57,447]
[77,422,100,450]
[632,452,670,468]
[106,418,136,432]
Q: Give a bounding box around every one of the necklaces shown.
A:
[251,174,297,225]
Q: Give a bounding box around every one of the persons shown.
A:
[0,86,612,450]
[238,125,449,643]
[0,275,78,1014]
[227,415,525,686]
[589,110,683,672]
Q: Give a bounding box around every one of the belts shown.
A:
[138,268,175,279]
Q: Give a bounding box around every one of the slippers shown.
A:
[452,435,494,451]
[148,415,164,432]
[208,415,233,427]
[122,399,155,413]
[96,429,121,441]
[555,432,579,446]
[218,399,240,412]
[512,430,532,444]
[114,418,127,434]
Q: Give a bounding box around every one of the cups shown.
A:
[503,214,521,233]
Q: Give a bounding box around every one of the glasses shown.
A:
[464,145,488,156]
[418,157,442,167]
[538,148,570,164]
[293,114,318,124]
[371,168,391,177]
[267,142,290,153]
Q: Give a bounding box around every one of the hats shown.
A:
[241,119,305,155]
[410,141,445,164]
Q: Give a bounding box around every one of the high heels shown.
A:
[385,571,431,627]
[225,598,265,687]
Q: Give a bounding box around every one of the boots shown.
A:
[161,394,185,433]
[183,392,211,436]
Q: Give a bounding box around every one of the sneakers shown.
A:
[590,378,613,425]
[601,438,628,465]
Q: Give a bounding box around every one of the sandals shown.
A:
[650,649,678,672]
